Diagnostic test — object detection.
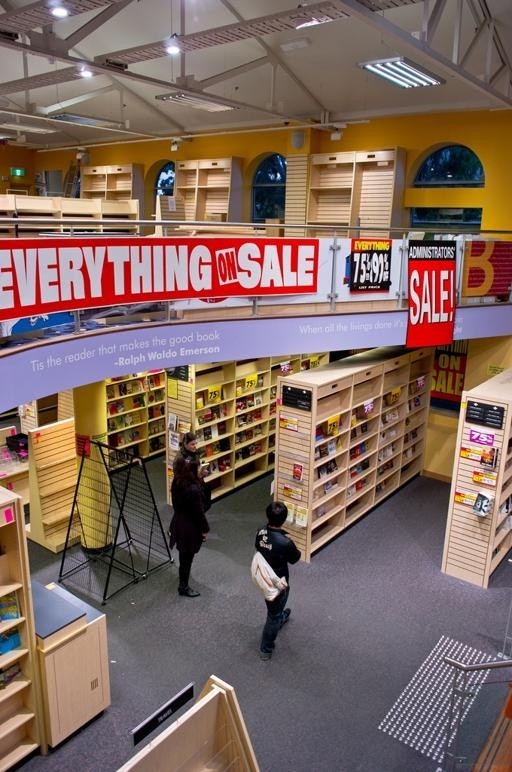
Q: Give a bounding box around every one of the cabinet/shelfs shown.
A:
[32,580,112,750]
[0,426,30,504]
[1,484,49,772]
[71,367,166,472]
[25,417,81,553]
[1,145,408,239]
[21,393,58,436]
[164,352,331,506]
[273,343,436,563]
[114,673,260,771]
[439,366,511,591]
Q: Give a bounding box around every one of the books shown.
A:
[1,590,20,620]
[1,660,23,687]
[105,370,165,466]
[198,377,277,475]
[312,379,419,517]
[0,624,21,655]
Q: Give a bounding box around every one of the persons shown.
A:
[167,458,212,596]
[171,431,209,542]
[254,502,302,660]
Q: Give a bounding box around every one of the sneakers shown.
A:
[260,641,275,661]
[281,607,291,628]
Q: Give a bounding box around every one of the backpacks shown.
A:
[250,551,288,601]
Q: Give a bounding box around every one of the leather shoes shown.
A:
[178,585,201,598]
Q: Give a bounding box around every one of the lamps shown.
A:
[155,1,240,114]
[48,62,125,129]
[355,9,446,90]
